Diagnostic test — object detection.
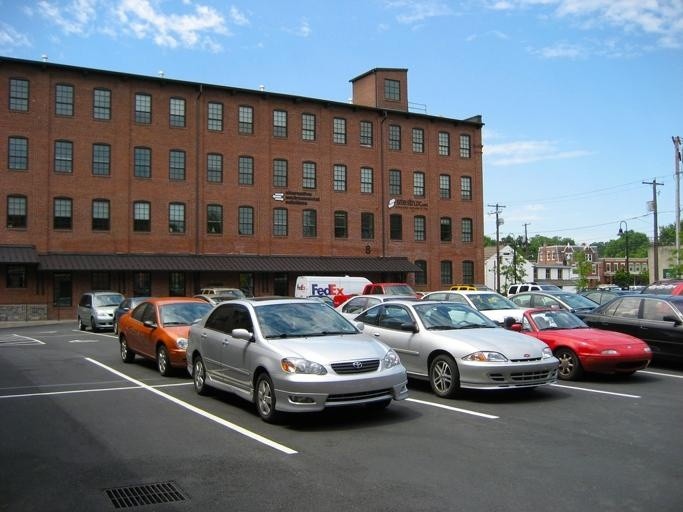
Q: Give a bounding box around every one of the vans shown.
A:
[295,277,374,298]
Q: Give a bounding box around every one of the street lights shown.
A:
[509,240,521,283]
[616,219,631,276]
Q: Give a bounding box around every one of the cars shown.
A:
[421,291,552,328]
[74,289,126,331]
[507,279,683,310]
[117,296,213,379]
[198,286,245,297]
[415,291,429,297]
[346,301,559,398]
[362,282,417,299]
[184,296,409,422]
[190,294,238,306]
[571,292,682,369]
[110,296,152,334]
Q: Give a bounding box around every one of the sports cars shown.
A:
[491,306,654,381]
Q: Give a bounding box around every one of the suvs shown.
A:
[450,284,478,292]
[505,282,564,296]
